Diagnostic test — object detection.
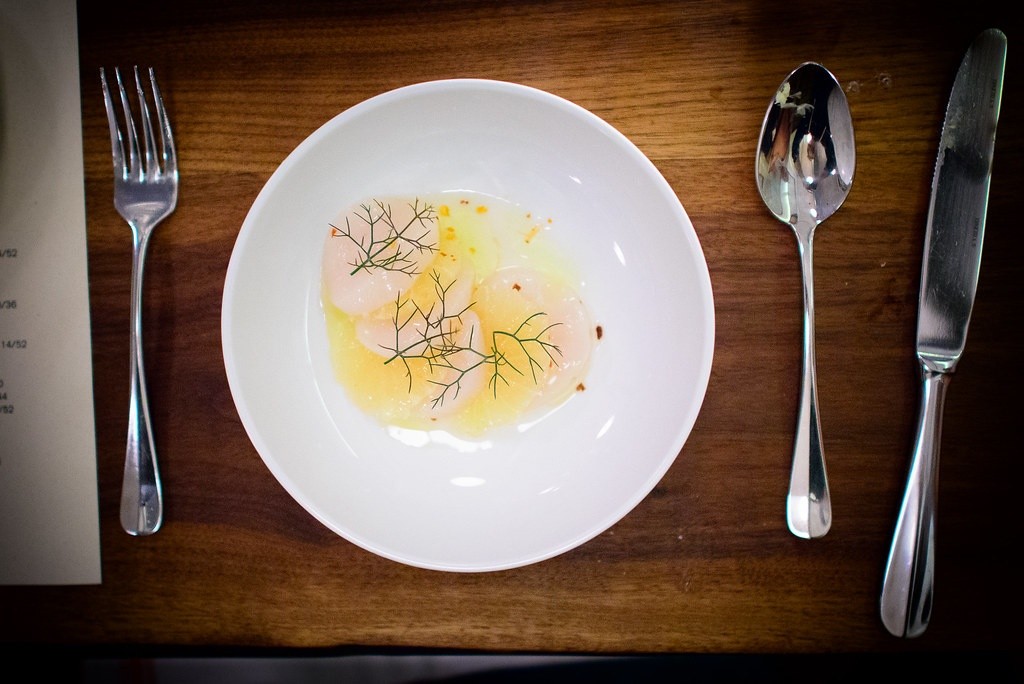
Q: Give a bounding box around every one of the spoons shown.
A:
[754,61,857,541]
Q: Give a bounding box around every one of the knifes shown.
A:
[877,28,1010,637]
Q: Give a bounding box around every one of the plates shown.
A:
[219,79,716,572]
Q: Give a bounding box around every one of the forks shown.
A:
[97,65,179,538]
